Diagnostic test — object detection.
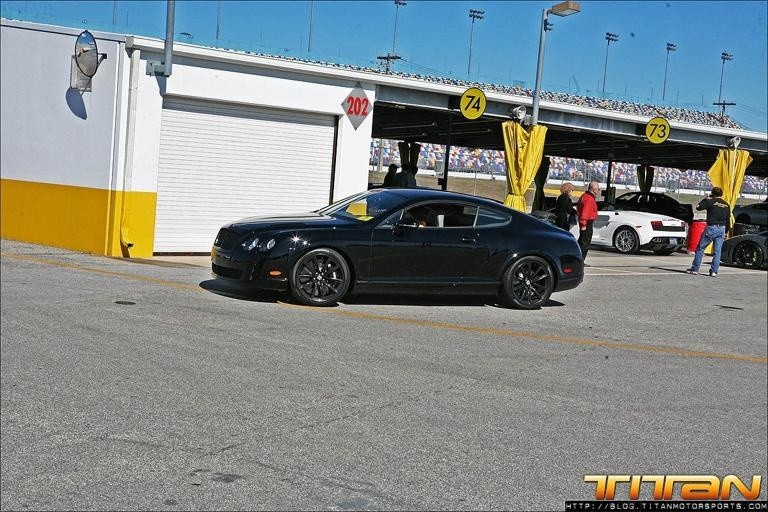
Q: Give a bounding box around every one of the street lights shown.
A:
[468,9,485,74]
[393,0,407,56]
[524,0,580,214]
[663,42,677,100]
[603,32,619,98]
[719,51,734,103]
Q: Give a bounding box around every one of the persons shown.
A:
[576,181,600,267]
[368,135,768,196]
[556,182,577,232]
[686,187,731,277]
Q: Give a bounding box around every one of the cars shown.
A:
[211,187,583,310]
[528,192,768,271]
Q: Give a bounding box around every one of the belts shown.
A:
[708,224,725,227]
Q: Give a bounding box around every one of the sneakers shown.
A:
[686,268,718,277]
